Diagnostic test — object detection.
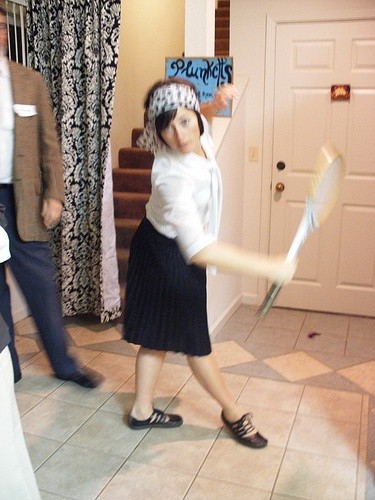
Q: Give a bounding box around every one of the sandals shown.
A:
[128,408,183,429]
[222,409,268,448]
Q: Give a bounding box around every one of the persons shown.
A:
[1,8,106,389]
[122,77,268,452]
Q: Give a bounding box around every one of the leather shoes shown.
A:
[53,365,102,389]
